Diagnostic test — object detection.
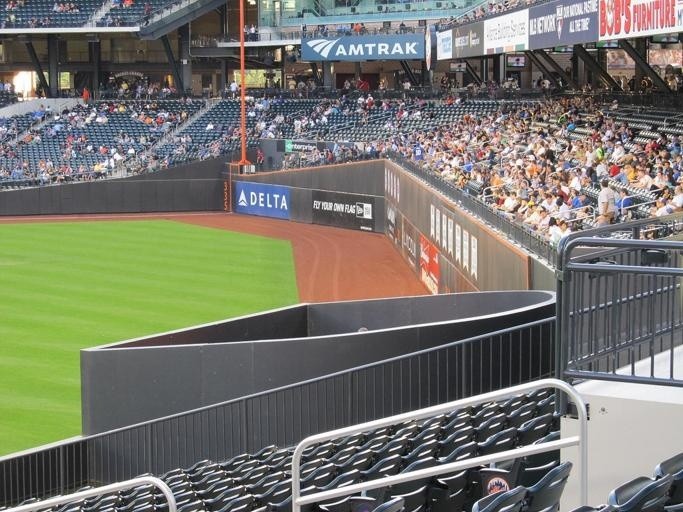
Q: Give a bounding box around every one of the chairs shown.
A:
[219,386,570,511]
[60,457,258,511]
[574,450,682,510]
